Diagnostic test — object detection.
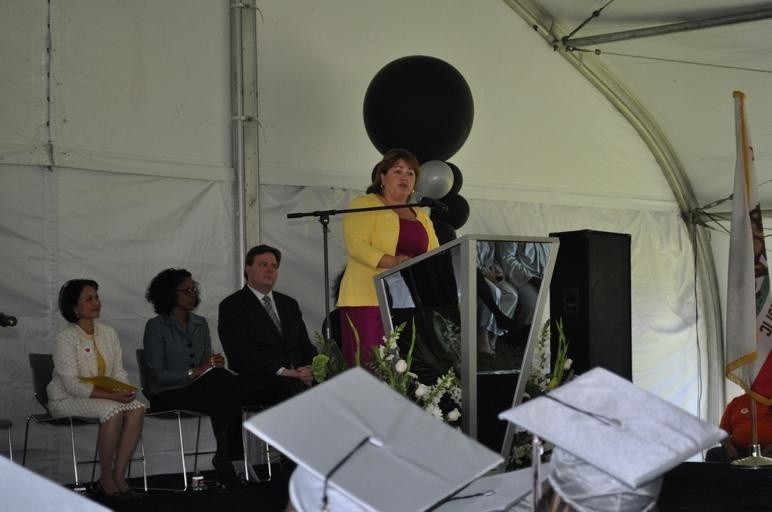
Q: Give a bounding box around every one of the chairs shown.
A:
[21,347,212,492]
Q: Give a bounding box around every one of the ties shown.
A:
[262,295,283,335]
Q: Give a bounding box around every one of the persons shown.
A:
[704,391,772,463]
[475,239,552,365]
[321,262,347,353]
[141,267,248,492]
[218,242,329,478]
[46,280,146,502]
[337,149,441,370]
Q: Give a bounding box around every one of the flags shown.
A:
[726,95,771,404]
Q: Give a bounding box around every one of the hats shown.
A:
[240,366,507,512]
[498,366,729,512]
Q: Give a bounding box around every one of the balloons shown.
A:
[366,159,470,247]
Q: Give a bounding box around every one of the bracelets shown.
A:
[188,368,194,378]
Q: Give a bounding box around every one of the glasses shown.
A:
[177,286,198,296]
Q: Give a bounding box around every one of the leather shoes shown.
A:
[213,453,245,490]
[97,478,146,502]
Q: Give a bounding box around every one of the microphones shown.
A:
[410,191,449,212]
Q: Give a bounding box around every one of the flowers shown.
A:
[372,319,463,430]
[505,315,578,472]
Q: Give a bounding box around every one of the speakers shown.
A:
[549,229,632,386]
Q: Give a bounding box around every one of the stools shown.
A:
[240,404,275,484]
